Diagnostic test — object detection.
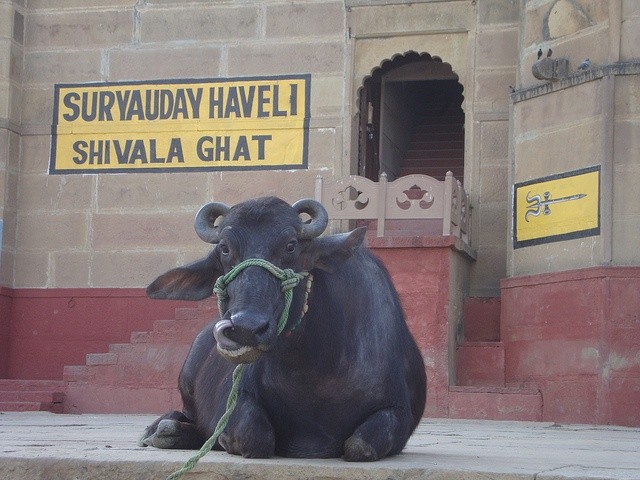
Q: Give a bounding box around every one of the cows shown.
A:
[137,196,427,461]
